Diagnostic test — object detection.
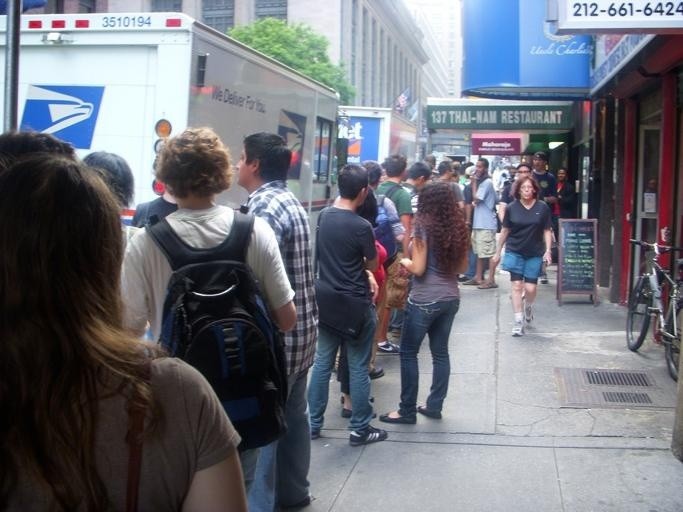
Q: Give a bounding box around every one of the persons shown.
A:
[0,128,78,165]
[0,155,250,512]
[119,126,299,498]
[306,163,390,446]
[235,130,321,511]
[82,151,134,208]
[379,180,470,424]
[332,152,576,419]
[133,189,178,229]
[489,171,554,337]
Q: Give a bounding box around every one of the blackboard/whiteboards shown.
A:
[558,218,598,294]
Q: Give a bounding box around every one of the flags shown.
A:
[408,98,418,120]
[393,85,413,114]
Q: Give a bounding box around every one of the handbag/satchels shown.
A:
[384,273,409,310]
[312,278,369,339]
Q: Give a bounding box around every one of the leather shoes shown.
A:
[379,411,415,424]
[415,404,441,418]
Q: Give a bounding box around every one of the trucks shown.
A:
[1,11,342,246]
[337,106,416,180]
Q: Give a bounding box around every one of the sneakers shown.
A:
[459,277,498,289]
[523,298,533,324]
[375,341,400,355]
[341,408,376,419]
[369,367,383,378]
[310,430,319,438]
[340,395,374,405]
[512,320,522,336]
[349,425,387,446]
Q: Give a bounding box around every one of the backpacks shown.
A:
[145,205,287,454]
[372,195,396,269]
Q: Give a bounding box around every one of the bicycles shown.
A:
[625,236,683,379]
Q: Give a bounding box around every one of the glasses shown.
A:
[517,170,532,174]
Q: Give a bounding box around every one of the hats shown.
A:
[534,151,549,161]
